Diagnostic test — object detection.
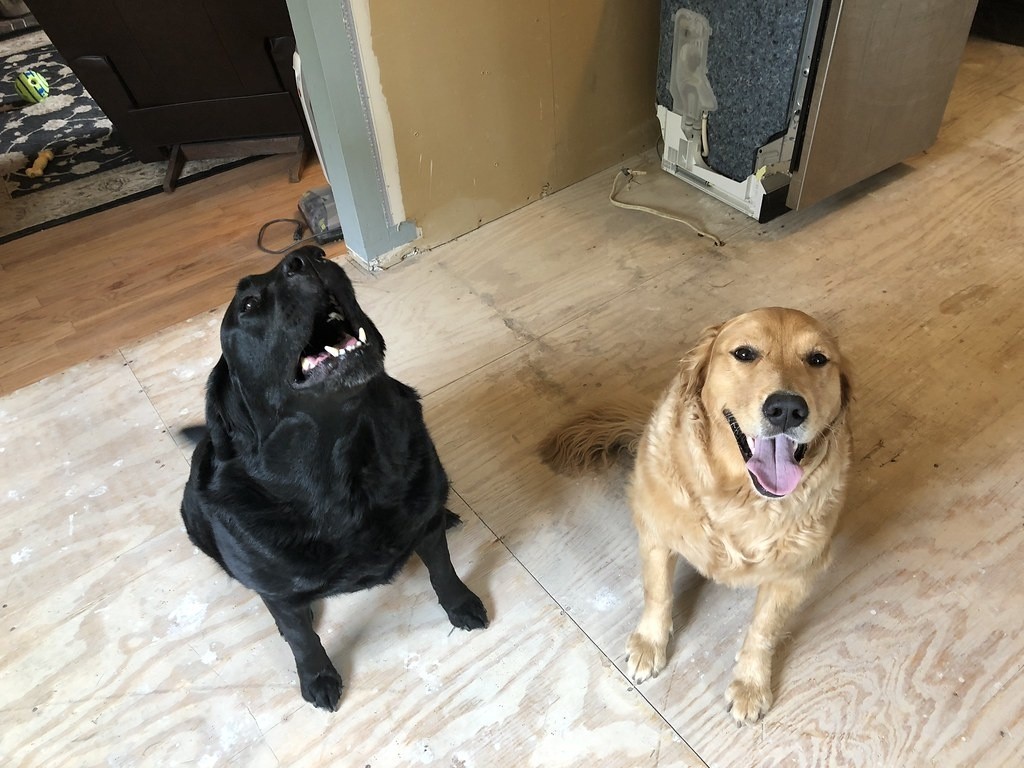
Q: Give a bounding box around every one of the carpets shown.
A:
[1,23,281,244]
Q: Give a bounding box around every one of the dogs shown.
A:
[178,245,489,711]
[535,306,856,729]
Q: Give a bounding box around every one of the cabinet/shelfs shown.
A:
[23,0,311,193]
